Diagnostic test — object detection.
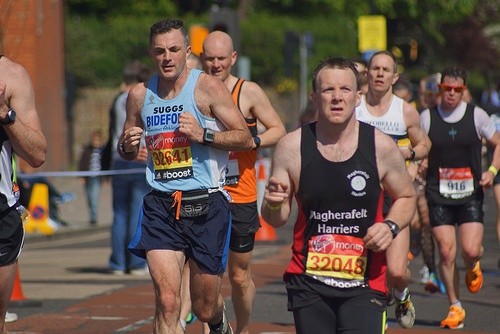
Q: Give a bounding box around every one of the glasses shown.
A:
[439,84,465,93]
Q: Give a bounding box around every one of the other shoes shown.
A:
[112,269,124,275]
[130,269,146,276]
[5,311,18,323]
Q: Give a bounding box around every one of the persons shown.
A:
[77,128,106,224]
[17,164,76,227]
[262,57,418,334]
[0,54,46,334]
[118,19,287,334]
[354,51,500,329]
[107,67,148,276]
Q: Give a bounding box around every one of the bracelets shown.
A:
[488,166,497,176]
[120,143,130,155]
[266,202,283,210]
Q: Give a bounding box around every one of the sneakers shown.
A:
[425,271,446,296]
[464,260,483,294]
[207,301,234,334]
[440,305,465,329]
[418,265,429,285]
[394,289,415,329]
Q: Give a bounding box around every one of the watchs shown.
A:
[383,219,399,239]
[1,108,16,125]
[201,127,215,146]
[410,149,415,160]
[252,136,261,150]
